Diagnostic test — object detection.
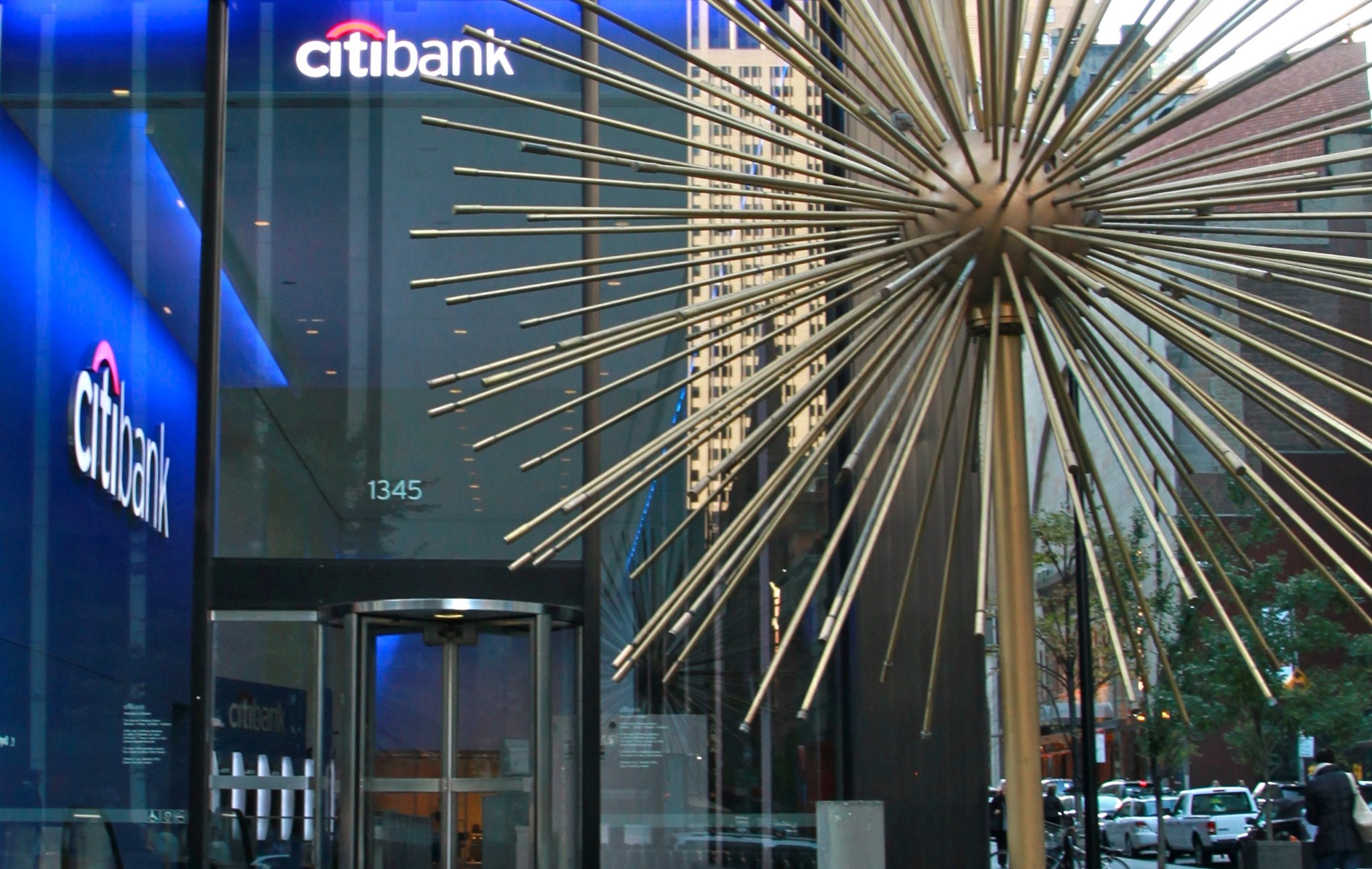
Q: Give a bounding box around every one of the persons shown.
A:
[1042,784,1063,832]
[427,811,483,869]
[1238,780,1249,789]
[1305,746,1368,869]
[990,779,1009,869]
[1212,780,1222,787]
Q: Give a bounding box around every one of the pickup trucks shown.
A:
[1161,786,1262,868]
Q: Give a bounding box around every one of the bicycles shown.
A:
[990,822,1130,869]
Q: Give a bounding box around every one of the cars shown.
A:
[1098,779,1153,798]
[1059,793,1124,845]
[988,787,1001,804]
[1099,796,1181,857]
[667,830,818,869]
[1253,779,1372,814]
[1041,777,1075,795]
[1228,796,1320,869]
